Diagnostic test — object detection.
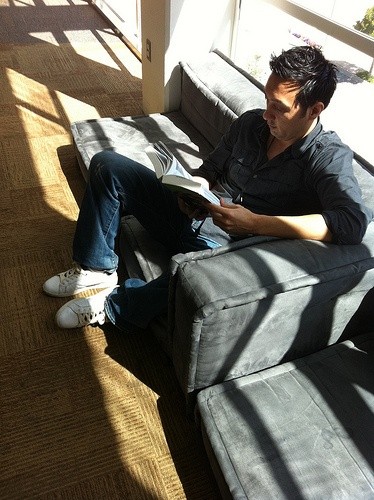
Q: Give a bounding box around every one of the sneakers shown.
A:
[55,285,121,329]
[42,265,118,297]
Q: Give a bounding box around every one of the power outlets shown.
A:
[146,38,151,62]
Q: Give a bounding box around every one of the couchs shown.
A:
[69,47,374,419]
[192,330,374,500]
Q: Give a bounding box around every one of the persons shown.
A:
[43,46,374,329]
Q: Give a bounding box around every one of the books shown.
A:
[143,140,222,217]
